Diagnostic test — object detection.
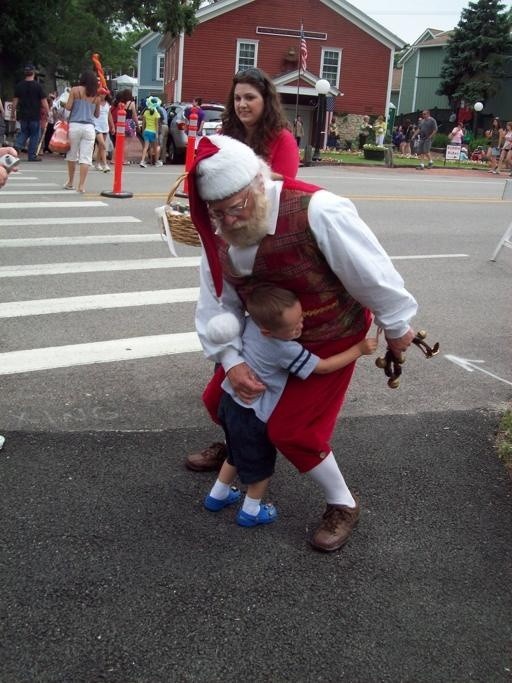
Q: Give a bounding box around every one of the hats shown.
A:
[195,133,259,200]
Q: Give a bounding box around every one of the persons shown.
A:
[293,114,305,149]
[0,66,205,191]
[358,109,438,169]
[183,135,419,555]
[203,282,377,529]
[218,68,299,180]
[447,117,512,177]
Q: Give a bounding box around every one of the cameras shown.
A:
[0,154,20,171]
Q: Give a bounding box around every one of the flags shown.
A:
[300,24,307,75]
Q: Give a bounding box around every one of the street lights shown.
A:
[473,101,484,138]
[310,78,331,161]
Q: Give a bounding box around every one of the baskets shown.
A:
[163,170,202,246]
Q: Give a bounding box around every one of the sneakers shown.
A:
[185,440,227,471]
[236,503,276,526]
[311,491,359,550]
[204,485,241,510]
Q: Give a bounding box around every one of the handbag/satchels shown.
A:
[48,120,70,153]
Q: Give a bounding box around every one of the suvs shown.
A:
[134,101,229,163]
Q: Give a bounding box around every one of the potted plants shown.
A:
[361,143,386,160]
